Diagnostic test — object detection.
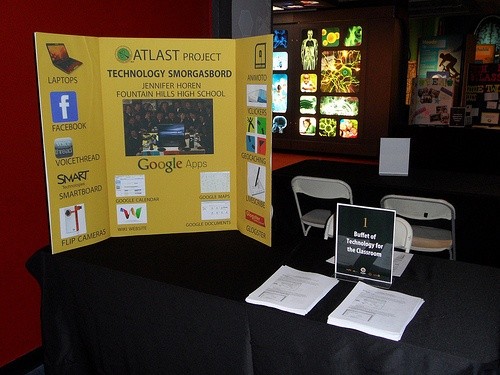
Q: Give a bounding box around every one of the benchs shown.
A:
[392,124,500,191]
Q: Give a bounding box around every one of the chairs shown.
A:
[291,176,354,241]
[380,194,456,259]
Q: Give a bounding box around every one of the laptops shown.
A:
[46,43,82,74]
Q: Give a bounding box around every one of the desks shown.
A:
[26,159,500,374]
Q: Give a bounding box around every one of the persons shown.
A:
[124,100,212,149]
[439,53,459,77]
